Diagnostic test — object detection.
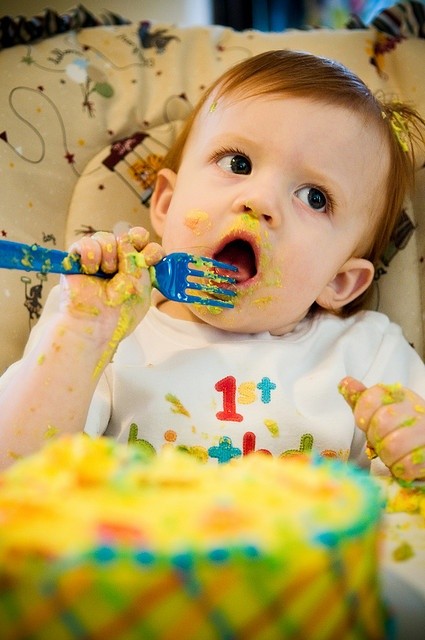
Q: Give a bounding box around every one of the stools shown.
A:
[1,29,424,388]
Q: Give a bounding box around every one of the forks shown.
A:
[0,239,239,311]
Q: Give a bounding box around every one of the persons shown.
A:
[0,48,425,488]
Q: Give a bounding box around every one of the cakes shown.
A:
[1,435,385,636]
